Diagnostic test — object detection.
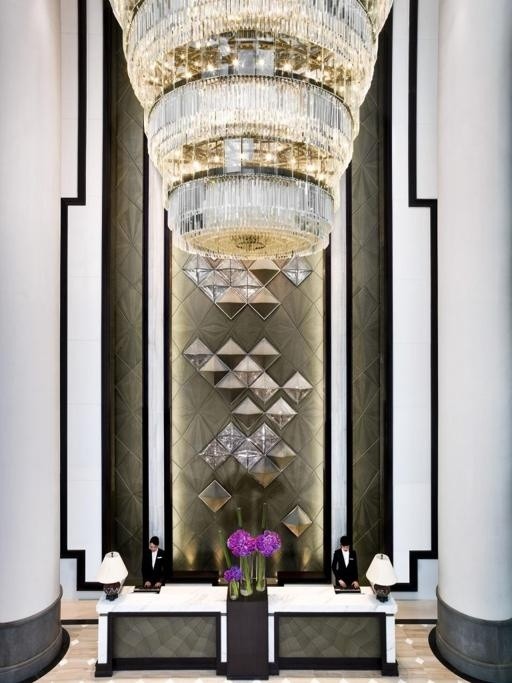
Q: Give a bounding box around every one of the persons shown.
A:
[141,535,168,588]
[332,535,360,589]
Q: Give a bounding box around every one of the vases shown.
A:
[231,555,269,600]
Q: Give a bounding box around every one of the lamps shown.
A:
[365,553,399,602]
[97,552,129,600]
[110,0,395,258]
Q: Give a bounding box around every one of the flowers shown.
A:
[224,527,282,582]
